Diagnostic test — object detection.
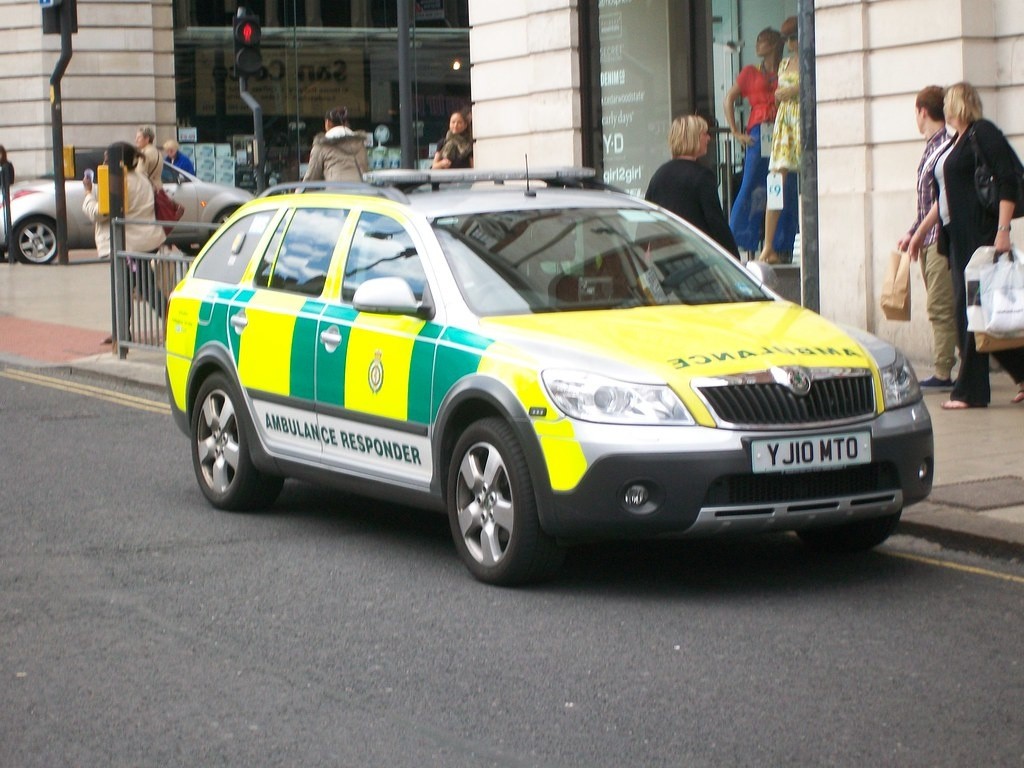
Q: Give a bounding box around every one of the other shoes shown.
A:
[101,332,132,345]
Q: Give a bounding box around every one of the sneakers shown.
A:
[918,374,952,392]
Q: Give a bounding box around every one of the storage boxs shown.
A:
[179,143,235,187]
[368,151,401,170]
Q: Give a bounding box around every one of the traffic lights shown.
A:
[233,16,262,76]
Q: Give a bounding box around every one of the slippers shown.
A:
[1011,389,1024,403]
[941,399,988,409]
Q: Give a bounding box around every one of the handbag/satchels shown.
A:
[963,243,1024,352]
[150,186,183,233]
[881,244,913,321]
[969,119,1023,218]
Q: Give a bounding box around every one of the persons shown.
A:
[82,141,167,346]
[757,15,801,267]
[723,28,800,265]
[643,114,742,264]
[896,84,965,393]
[134,126,162,189]
[162,140,196,183]
[908,81,1024,413]
[301,107,373,192]
[430,111,473,171]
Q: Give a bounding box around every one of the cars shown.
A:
[0,148,256,266]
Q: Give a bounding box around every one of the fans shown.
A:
[374,124,389,149]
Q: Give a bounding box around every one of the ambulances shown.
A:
[165,182,934,586]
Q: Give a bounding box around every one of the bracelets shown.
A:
[998,225,1012,231]
